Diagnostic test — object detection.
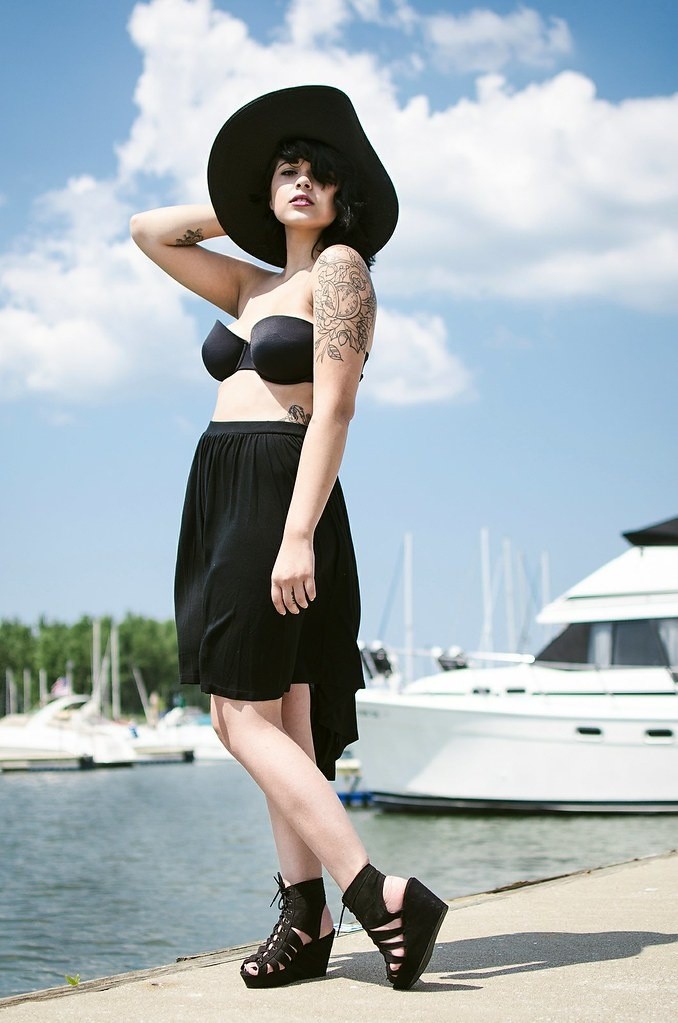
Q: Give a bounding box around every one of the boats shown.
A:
[352,516,678,819]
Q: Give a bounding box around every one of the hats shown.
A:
[207,86,399,269]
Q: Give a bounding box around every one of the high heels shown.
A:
[240,872,338,989]
[336,863,449,990]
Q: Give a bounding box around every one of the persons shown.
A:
[130,121,449,989]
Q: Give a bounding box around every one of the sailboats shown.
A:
[0,617,199,771]
